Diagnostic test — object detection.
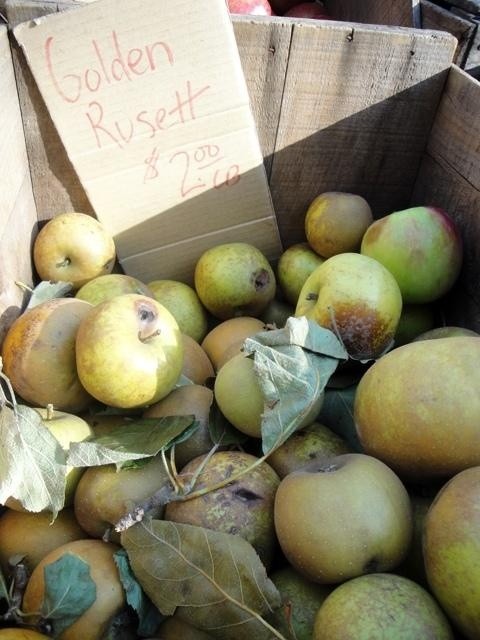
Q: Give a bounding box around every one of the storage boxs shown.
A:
[0,0,480,335]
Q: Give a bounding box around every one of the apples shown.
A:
[0,191,480,640]
